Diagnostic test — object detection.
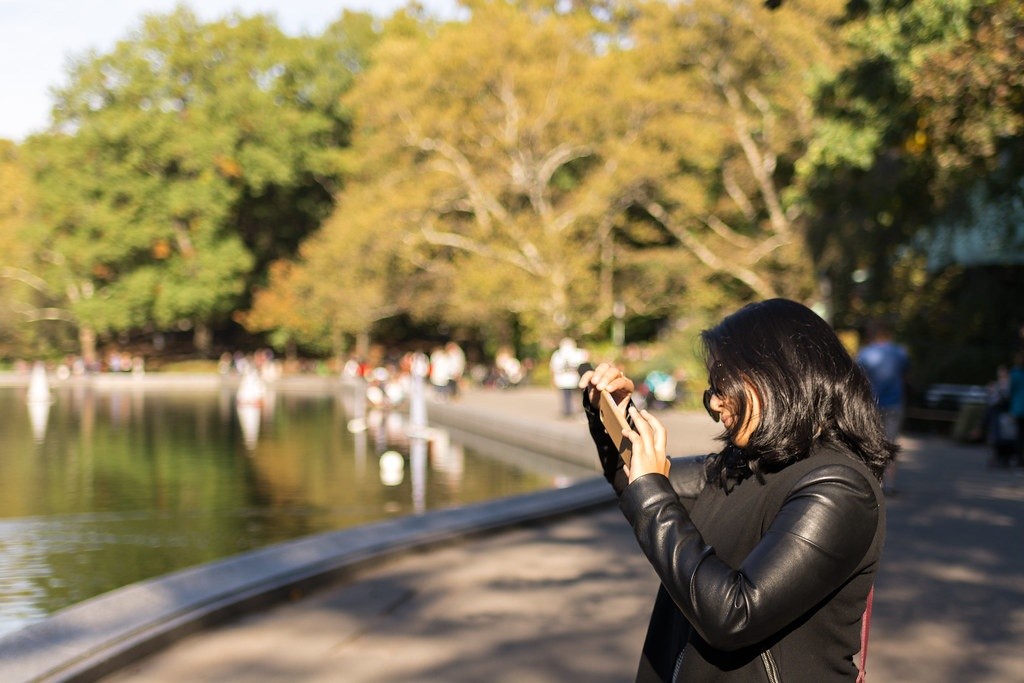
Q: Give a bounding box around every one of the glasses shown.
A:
[702,361,732,423]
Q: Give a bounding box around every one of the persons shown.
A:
[580,297,903,683]
[855,328,908,444]
[387,334,687,418]
[963,361,1024,468]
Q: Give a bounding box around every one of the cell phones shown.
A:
[599,389,634,468]
